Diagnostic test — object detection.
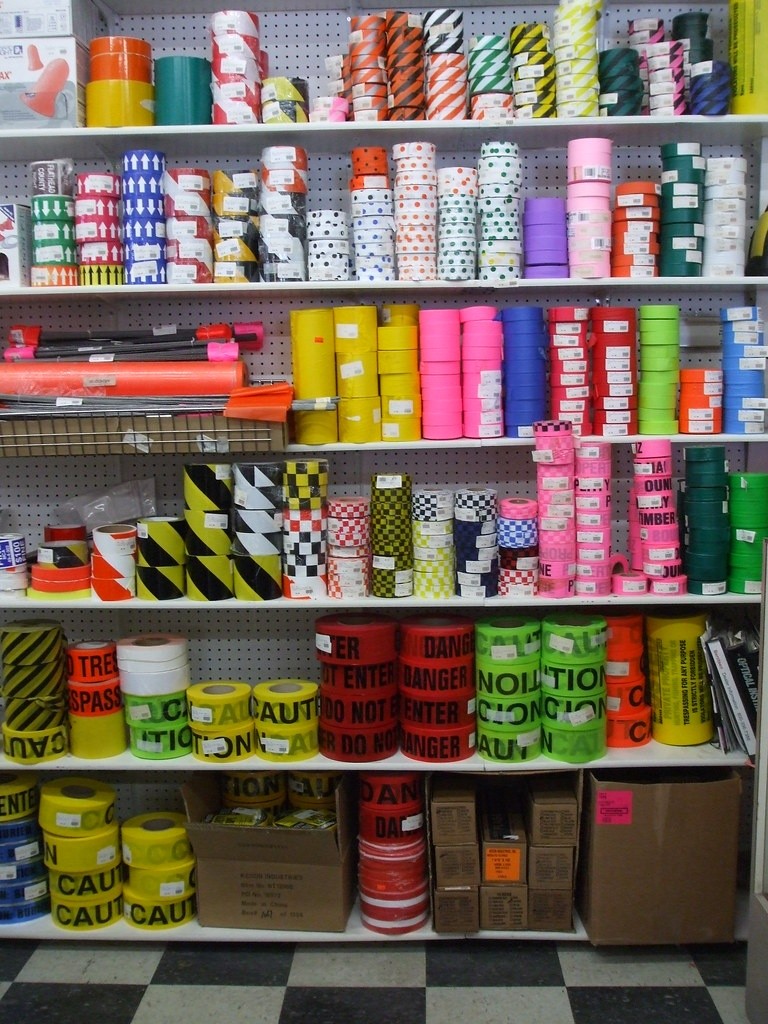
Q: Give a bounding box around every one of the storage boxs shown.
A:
[573,765,744,945]
[432,886,479,933]
[528,886,573,930]
[527,777,577,848]
[435,843,480,886]
[183,771,358,931]
[0,38,90,129]
[527,842,574,889]
[431,778,477,845]
[423,769,586,932]
[0,0,97,50]
[477,791,527,884]
[478,884,528,929]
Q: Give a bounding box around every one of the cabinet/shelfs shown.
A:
[0,0,768,942]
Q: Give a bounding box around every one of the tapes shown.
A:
[292,301,768,436]
[1,422,768,599]
[28,138,748,276]
[0,613,710,762]
[86,0,732,121]
[0,773,427,932]
